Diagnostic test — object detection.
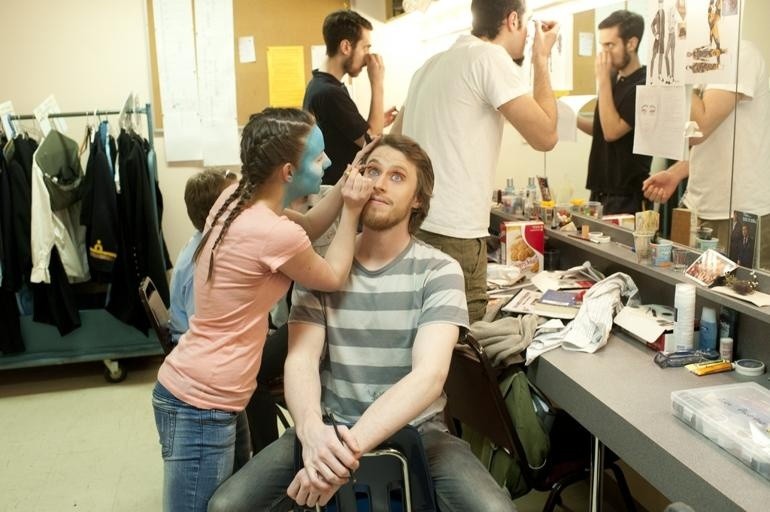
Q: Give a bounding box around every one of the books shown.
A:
[536,289,580,316]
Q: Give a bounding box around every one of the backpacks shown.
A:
[458,354,550,501]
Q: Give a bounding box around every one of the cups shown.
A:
[692,226,719,254]
[633,231,673,267]
[543,249,562,269]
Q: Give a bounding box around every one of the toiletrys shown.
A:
[696,306,717,356]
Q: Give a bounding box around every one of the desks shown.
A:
[521,286,770,511]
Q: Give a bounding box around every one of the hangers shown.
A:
[77,111,102,158]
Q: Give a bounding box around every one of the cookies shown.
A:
[511,235,539,273]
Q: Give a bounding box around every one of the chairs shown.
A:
[443,327,630,511]
[290,421,442,509]
[134,273,175,356]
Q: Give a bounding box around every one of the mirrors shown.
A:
[516,0,740,256]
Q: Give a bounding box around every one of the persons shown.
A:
[729,211,753,269]
[152,107,382,512]
[303,8,399,258]
[640,37,769,270]
[649,0,677,85]
[389,0,560,324]
[573,10,653,214]
[208,133,519,512]
[173,167,280,457]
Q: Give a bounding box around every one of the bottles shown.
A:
[672,283,695,353]
[699,305,717,352]
